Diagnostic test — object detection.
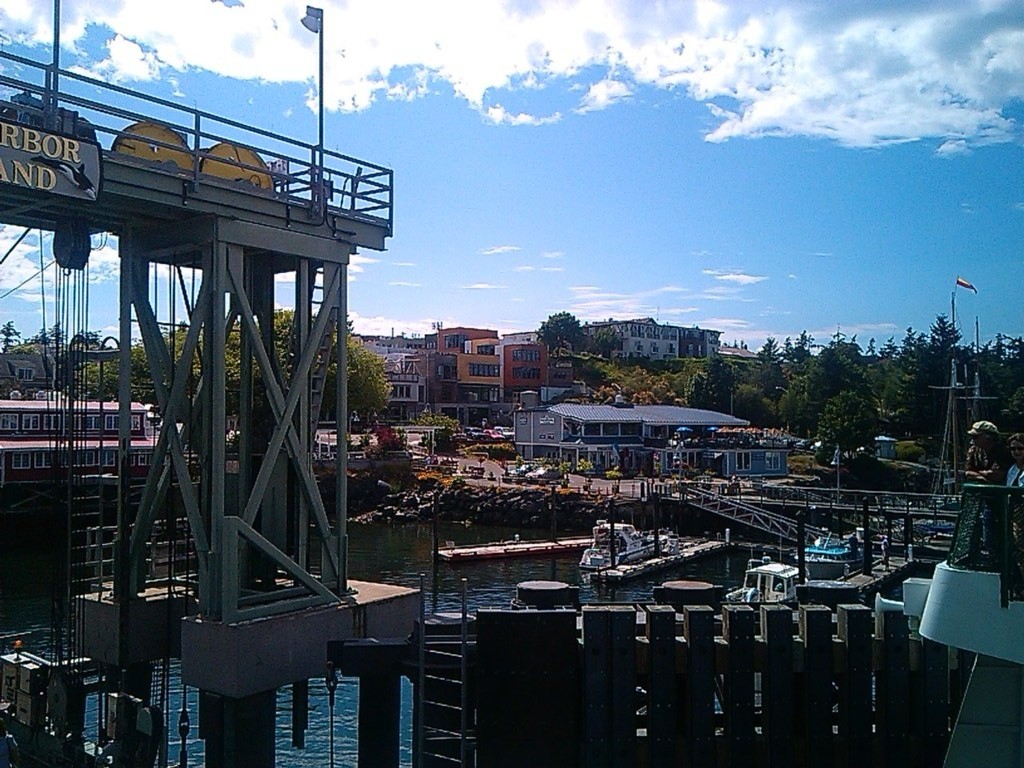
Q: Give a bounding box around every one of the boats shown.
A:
[579,521,670,575]
[795,537,865,581]
[932,521,957,532]
[726,562,808,609]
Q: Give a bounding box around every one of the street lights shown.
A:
[423,403,432,418]
[302,6,325,205]
[830,451,840,505]
[776,386,791,433]
[365,407,378,441]
[721,363,734,415]
[673,451,681,504]
[612,383,621,394]
[347,410,360,448]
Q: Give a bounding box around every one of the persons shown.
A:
[949,420,1024,601]
[849,532,858,561]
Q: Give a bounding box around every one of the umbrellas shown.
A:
[675,426,783,440]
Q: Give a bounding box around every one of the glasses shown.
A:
[1008,446,1024,451]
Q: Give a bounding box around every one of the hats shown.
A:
[966,421,1001,436]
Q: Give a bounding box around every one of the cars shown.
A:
[526,466,561,484]
[796,440,809,448]
[452,425,514,442]
[509,463,538,477]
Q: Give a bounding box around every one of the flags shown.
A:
[957,277,977,295]
